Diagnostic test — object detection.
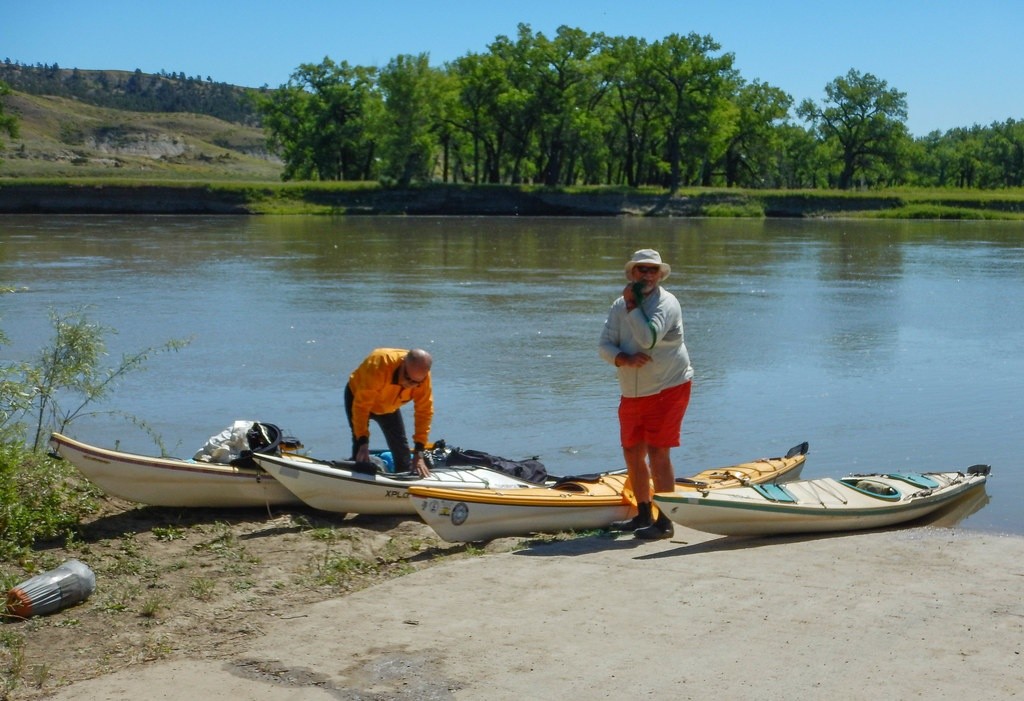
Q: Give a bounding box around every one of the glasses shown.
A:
[404,362,420,385]
[633,264,660,273]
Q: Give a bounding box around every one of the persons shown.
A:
[599,249,696,540]
[344,347,435,479]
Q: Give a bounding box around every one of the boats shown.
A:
[50,432,307,511]
[407,437,810,544]
[251,449,558,515]
[652,464,992,538]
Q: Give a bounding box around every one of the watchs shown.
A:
[413,448,424,459]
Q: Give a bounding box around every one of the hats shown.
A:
[625,248,671,282]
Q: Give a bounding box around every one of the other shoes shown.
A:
[634,518,674,539]
[611,515,654,531]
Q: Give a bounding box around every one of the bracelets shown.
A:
[625,298,636,305]
